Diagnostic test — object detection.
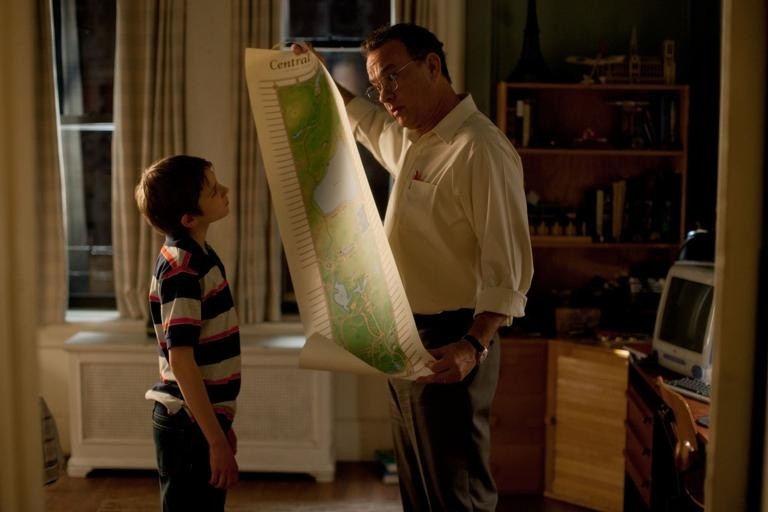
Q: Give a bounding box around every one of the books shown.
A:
[505,99,530,148]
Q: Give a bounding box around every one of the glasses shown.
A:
[364,59,415,102]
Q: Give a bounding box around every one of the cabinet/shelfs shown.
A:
[489,81,687,497]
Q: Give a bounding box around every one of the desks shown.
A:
[618,340,711,512]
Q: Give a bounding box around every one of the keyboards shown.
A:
[666,376,711,405]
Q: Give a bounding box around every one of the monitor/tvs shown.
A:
[652,260,714,384]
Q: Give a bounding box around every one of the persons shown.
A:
[135,155,241,512]
[283,23,534,512]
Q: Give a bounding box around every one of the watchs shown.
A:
[464,334,488,365]
